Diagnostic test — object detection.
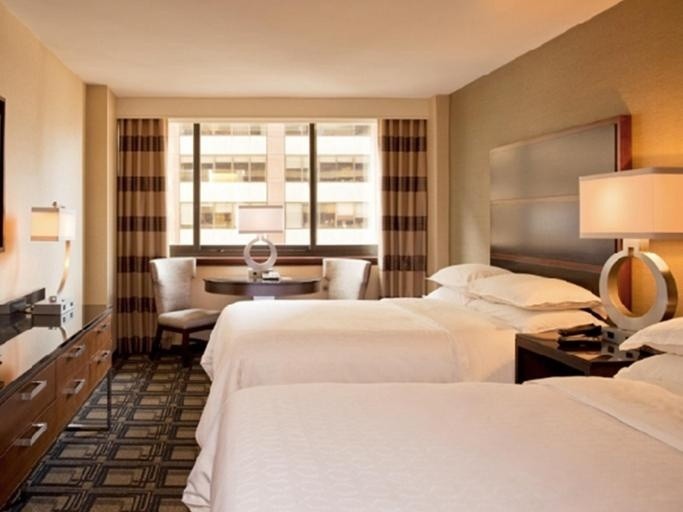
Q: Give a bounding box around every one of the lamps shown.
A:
[577,166,683,347]
[236,204,287,279]
[30,200,83,318]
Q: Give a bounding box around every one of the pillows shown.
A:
[611,353,683,399]
[418,263,513,286]
[464,271,608,322]
[418,286,477,303]
[616,315,683,355]
[464,298,609,336]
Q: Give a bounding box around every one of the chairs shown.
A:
[318,257,374,300]
[147,257,221,366]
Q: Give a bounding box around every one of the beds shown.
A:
[176,356,682,512]
[198,117,625,400]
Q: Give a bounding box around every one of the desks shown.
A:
[201,275,321,302]
[0,300,114,509]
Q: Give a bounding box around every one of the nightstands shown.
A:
[512,328,667,384]
